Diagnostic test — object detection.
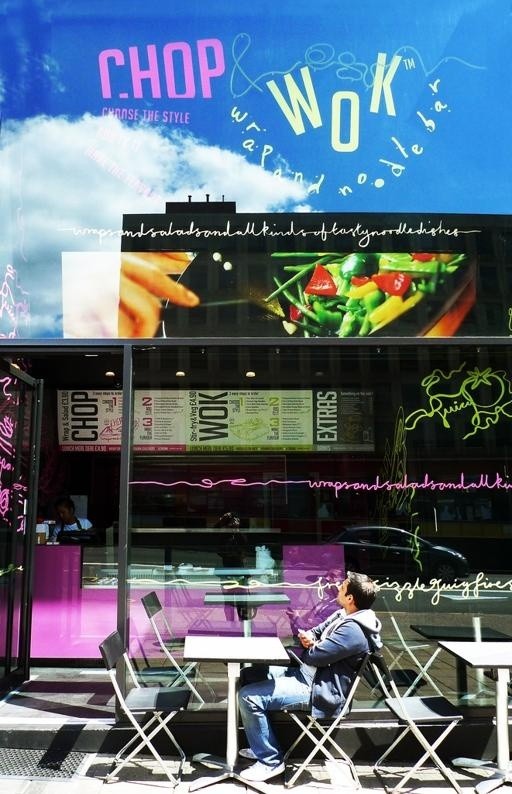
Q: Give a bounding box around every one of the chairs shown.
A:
[168,568,216,632]
[128,618,196,687]
[283,648,374,787]
[140,591,215,705]
[102,633,192,784]
[372,652,463,794]
[369,595,447,699]
[365,665,426,708]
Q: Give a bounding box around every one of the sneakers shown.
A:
[239,762,285,783]
[238,745,258,759]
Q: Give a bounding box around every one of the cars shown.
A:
[322,524,470,581]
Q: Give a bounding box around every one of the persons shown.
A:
[213,512,252,623]
[61,255,201,337]
[50,497,93,545]
[237,571,383,782]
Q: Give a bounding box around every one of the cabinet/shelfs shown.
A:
[80,543,285,659]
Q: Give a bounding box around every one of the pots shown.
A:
[160,251,484,338]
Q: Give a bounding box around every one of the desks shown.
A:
[434,594,510,700]
[188,621,278,636]
[409,624,512,706]
[437,641,512,793]
[183,636,291,794]
[205,592,291,670]
